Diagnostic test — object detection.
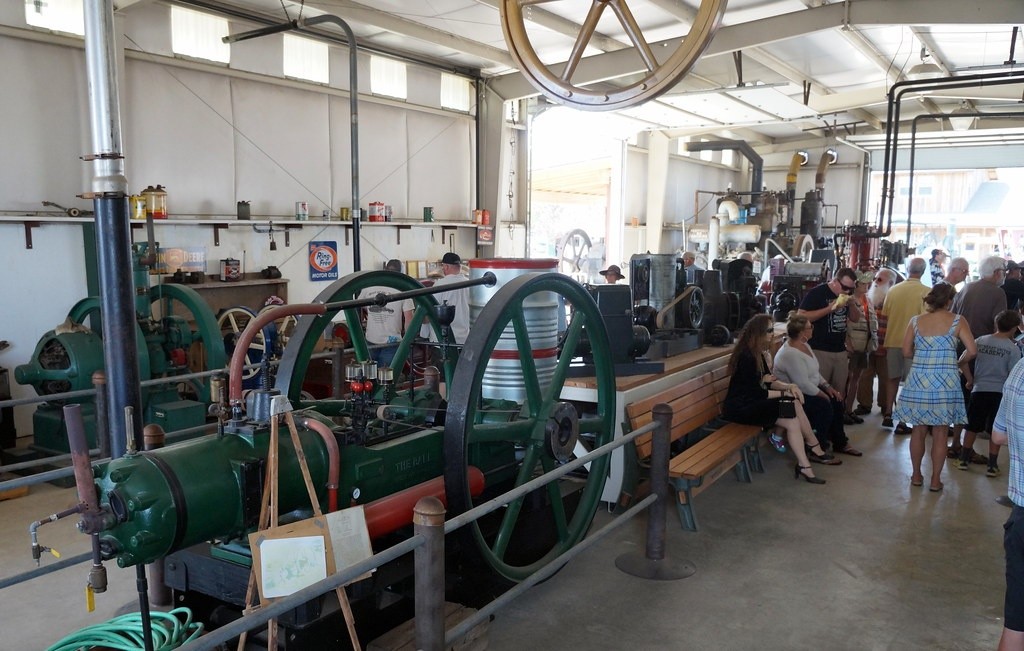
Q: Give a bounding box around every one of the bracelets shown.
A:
[781,391,784,399]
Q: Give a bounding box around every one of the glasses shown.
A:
[873,276,883,282]
[956,267,969,274]
[767,327,775,334]
[806,324,815,330]
[837,278,855,292]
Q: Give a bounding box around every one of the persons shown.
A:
[679,252,706,285]
[420,252,470,401]
[601,265,625,284]
[845,247,1024,476]
[797,266,860,410]
[773,314,864,464]
[356,259,415,390]
[736,253,755,266]
[898,279,978,492]
[725,315,842,484]
[755,254,786,296]
[991,357,1024,651]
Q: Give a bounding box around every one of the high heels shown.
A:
[794,463,826,484]
[806,443,833,458]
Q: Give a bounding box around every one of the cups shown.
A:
[341,208,348,220]
[360,208,367,221]
[323,210,330,220]
[129,194,146,219]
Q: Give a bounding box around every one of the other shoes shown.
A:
[843,411,865,424]
[882,416,894,427]
[808,451,842,465]
[948,443,988,463]
[834,443,862,456]
[895,422,913,434]
[854,404,872,414]
[768,433,785,452]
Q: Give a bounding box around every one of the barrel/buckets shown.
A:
[424,207,434,222]
[295,202,309,220]
[369,202,392,222]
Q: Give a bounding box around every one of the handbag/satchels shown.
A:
[778,391,796,418]
[848,349,867,368]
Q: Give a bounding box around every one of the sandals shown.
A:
[986,467,1001,476]
[953,457,968,470]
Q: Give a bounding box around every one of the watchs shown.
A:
[824,384,830,389]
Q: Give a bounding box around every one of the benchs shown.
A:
[626,365,764,533]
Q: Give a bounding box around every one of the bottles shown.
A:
[140,184,168,219]
[471,208,490,225]
[173,268,185,283]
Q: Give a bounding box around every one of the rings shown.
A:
[838,392,840,394]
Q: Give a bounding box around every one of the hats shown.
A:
[1006,259,1024,270]
[599,265,624,279]
[439,252,461,264]
[386,258,403,273]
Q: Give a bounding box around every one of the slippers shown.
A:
[930,481,944,491]
[910,473,924,485]
